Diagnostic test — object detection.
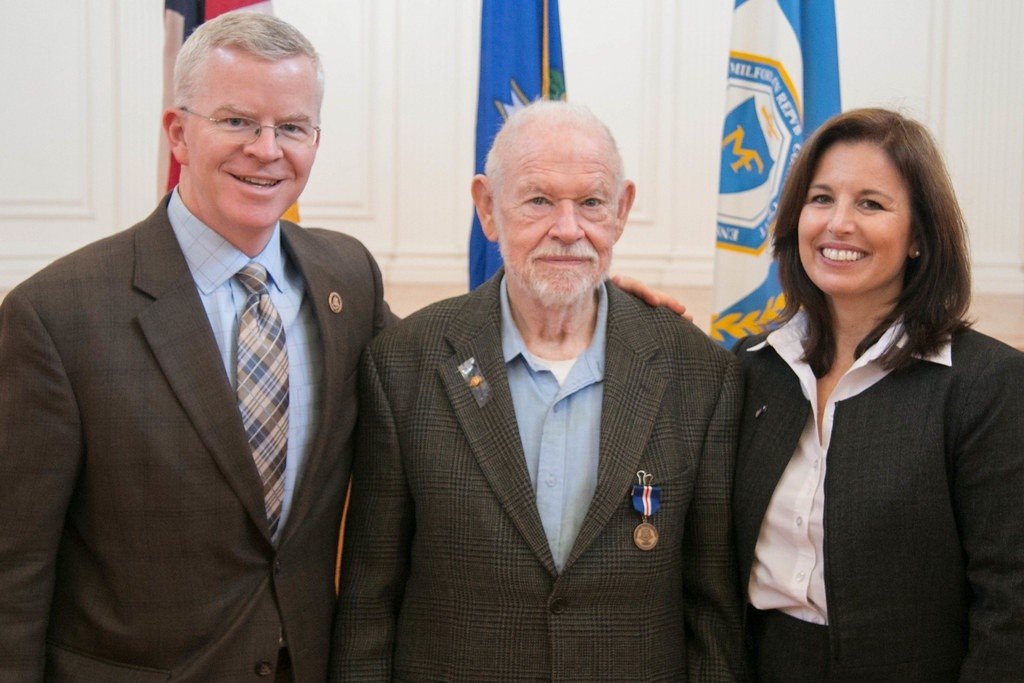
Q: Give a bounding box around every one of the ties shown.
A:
[236,264,289,549]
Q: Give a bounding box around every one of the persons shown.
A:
[732,108,1024,683]
[331,100,742,683]
[0,11,693,683]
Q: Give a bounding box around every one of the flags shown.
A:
[710,0,844,354]
[159,0,299,225]
[470,0,568,292]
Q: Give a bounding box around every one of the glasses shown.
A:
[177,105,321,150]
